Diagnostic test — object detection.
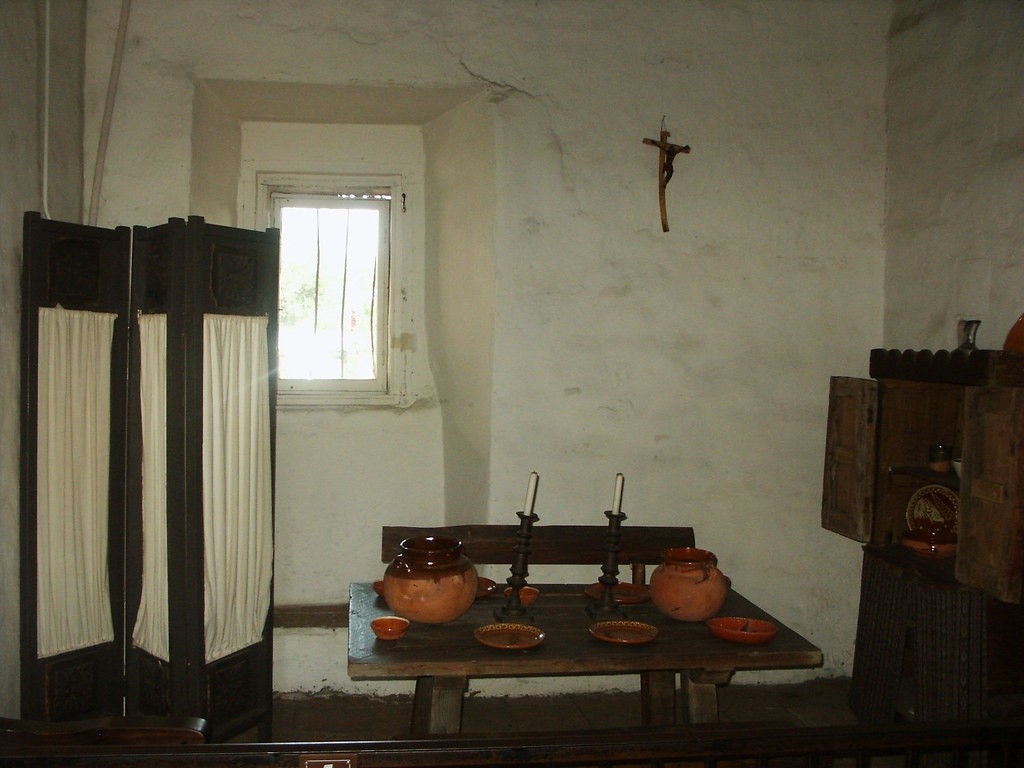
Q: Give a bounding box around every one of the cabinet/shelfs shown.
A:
[820,376,1024,601]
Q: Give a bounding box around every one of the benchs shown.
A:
[379,526,696,584]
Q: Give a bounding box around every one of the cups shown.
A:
[930,446,953,477]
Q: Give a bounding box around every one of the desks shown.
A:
[348,583,823,735]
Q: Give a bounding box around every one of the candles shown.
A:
[523,471,538,516]
[611,473,624,515]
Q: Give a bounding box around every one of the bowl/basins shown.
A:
[900,529,957,558]
[371,617,411,640]
[503,586,540,607]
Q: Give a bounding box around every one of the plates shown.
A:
[706,616,779,645]
[473,622,545,650]
[907,485,960,530]
[372,580,384,596]
[583,582,650,604]
[475,576,496,598]
[589,621,659,645]
